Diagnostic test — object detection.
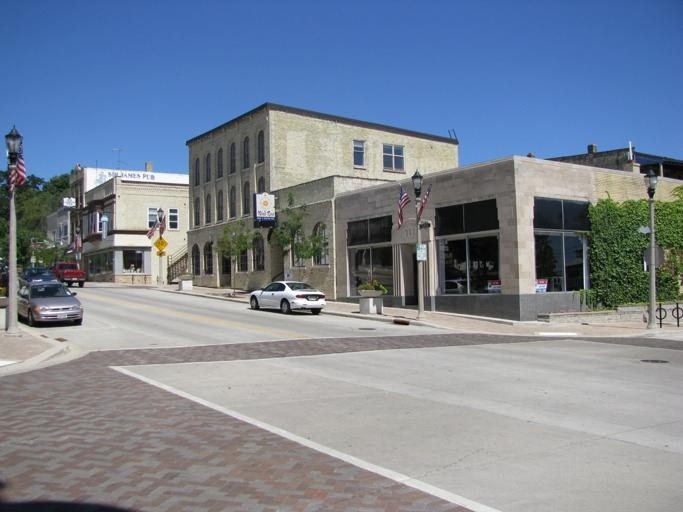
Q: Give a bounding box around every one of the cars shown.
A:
[21,267,57,286]
[249,279,326,314]
[442,279,470,294]
[17,281,83,326]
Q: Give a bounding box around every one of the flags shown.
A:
[5,137,27,200]
[146,219,159,240]
[415,185,432,224]
[159,217,167,240]
[397,186,412,232]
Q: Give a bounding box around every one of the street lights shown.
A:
[156,209,165,287]
[411,170,423,319]
[5,125,23,335]
[643,167,659,330]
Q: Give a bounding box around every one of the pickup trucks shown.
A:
[52,261,86,287]
